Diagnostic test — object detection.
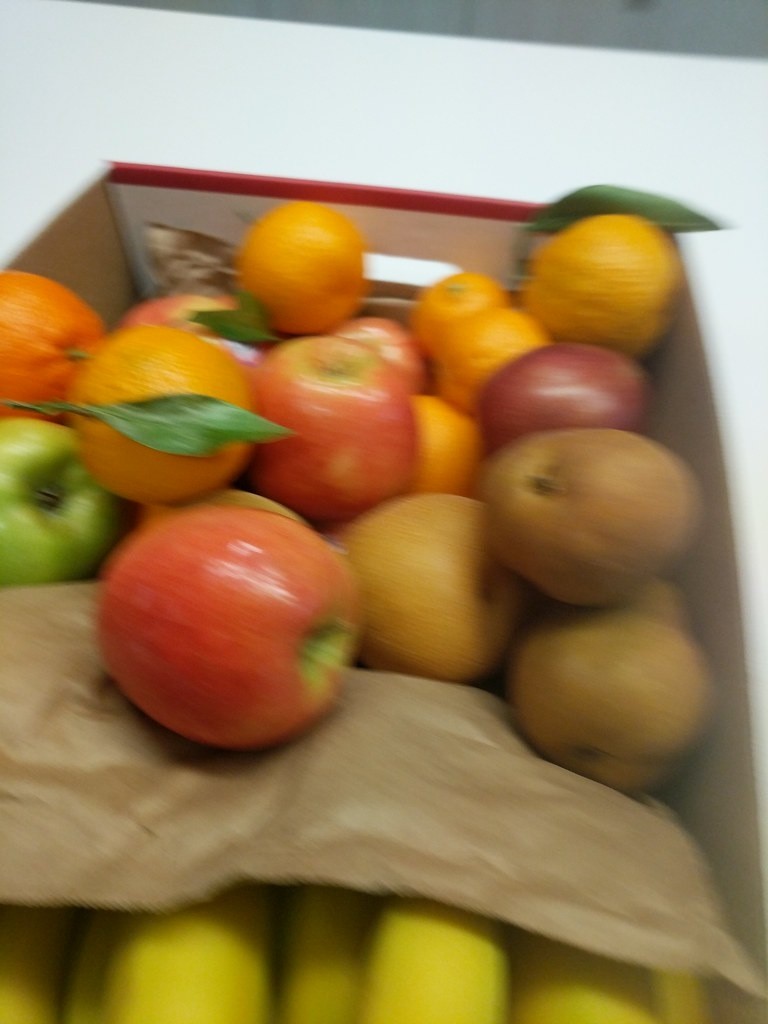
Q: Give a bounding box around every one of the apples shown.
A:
[0,293,653,750]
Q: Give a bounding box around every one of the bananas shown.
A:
[0,874,718,1024]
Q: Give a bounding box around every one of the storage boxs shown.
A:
[1,160,768,1024]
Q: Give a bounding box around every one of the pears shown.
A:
[328,432,712,794]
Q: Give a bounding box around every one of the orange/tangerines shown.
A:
[0,201,681,490]
